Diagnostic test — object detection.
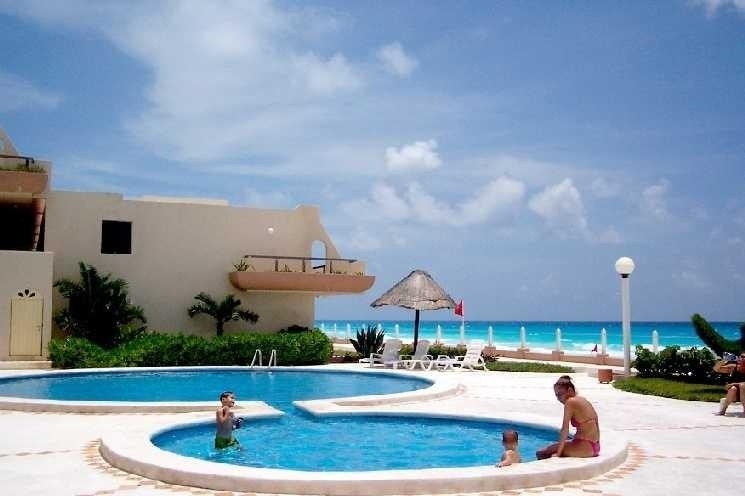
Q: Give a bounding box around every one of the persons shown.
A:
[713,353,745,415]
[215,392,236,451]
[536,375,601,458]
[495,430,519,467]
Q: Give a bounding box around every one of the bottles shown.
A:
[723,352,739,362]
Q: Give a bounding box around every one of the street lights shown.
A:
[616,257,636,376]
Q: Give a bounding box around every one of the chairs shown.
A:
[357,339,489,375]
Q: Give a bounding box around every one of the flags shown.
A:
[454,299,464,316]
